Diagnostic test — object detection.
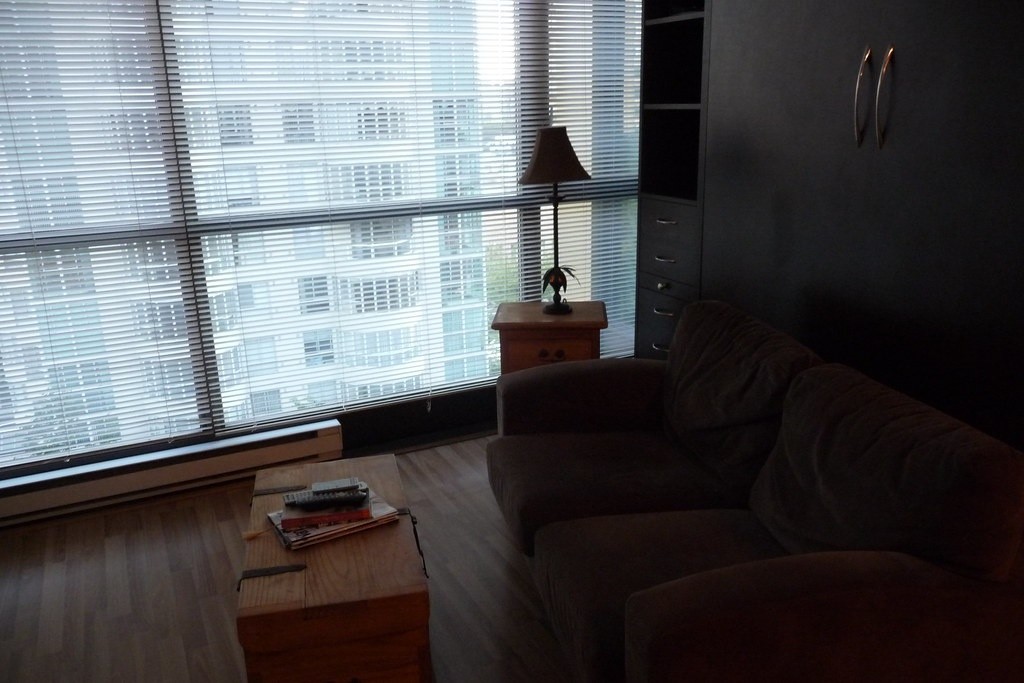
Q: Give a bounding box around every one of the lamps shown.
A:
[515,126,592,315]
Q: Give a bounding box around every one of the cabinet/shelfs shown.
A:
[632,1,1024,456]
[491,300,609,376]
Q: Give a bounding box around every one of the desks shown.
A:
[235,454,431,683]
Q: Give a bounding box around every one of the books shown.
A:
[266,476,400,549]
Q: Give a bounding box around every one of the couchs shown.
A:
[485,300,1024,683]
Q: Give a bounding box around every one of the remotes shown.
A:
[283,482,370,512]
